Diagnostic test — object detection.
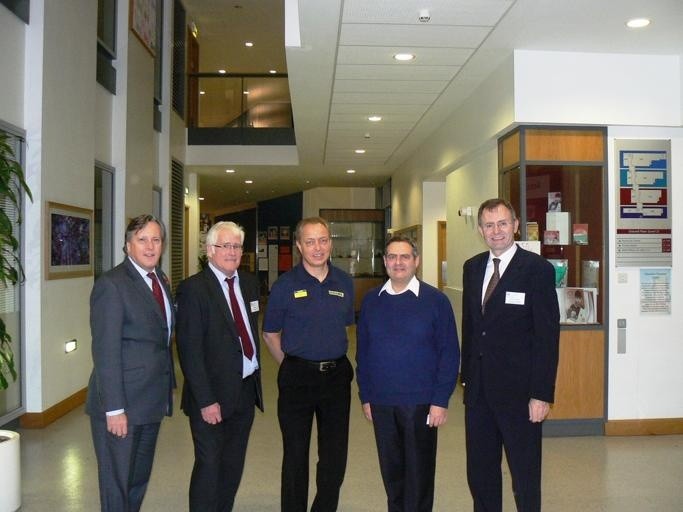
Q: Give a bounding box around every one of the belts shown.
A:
[285,355,346,373]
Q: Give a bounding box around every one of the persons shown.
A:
[352,234,461,511]
[171,219,266,511]
[261,216,357,511]
[566,291,585,320]
[454,197,561,512]
[83,213,178,512]
[549,194,560,211]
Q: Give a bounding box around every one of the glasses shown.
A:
[211,243,241,250]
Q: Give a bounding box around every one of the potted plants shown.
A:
[0,128,33,512]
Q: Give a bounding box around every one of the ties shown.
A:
[224,276,253,360]
[146,272,166,323]
[481,258,500,316]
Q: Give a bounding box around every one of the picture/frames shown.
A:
[40,199,94,282]
